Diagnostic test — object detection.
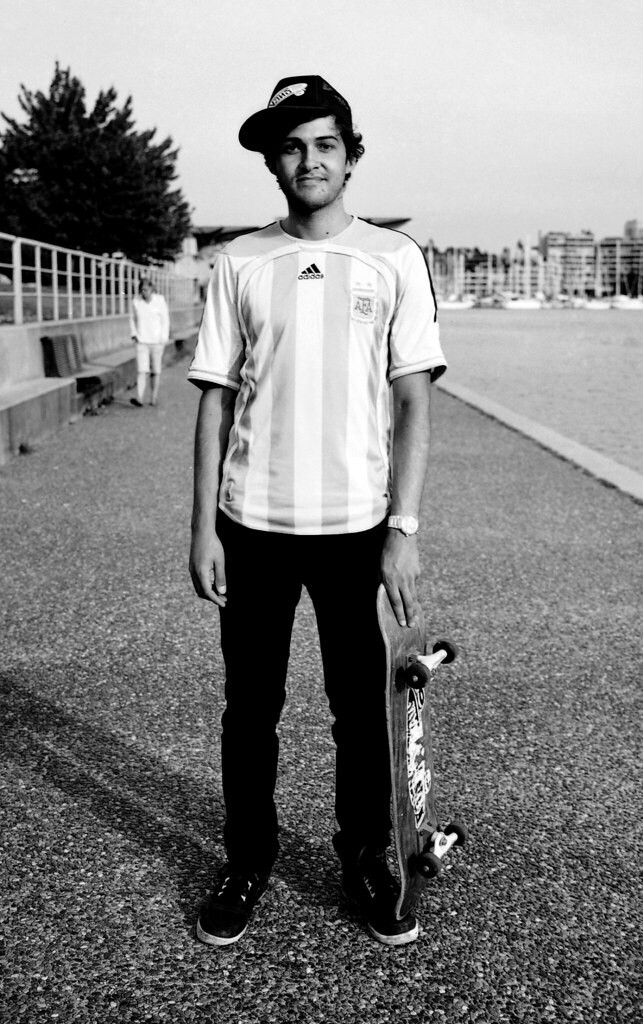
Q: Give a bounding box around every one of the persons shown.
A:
[188,75,448,947]
[130,279,169,407]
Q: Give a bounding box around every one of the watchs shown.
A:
[388,515,419,536]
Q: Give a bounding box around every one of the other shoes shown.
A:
[130,399,144,408]
[149,402,155,406]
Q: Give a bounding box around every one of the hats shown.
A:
[238,75,352,156]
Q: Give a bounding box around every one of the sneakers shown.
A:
[196,822,282,945]
[331,831,418,945]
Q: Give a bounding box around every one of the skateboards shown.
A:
[377,580,466,922]
